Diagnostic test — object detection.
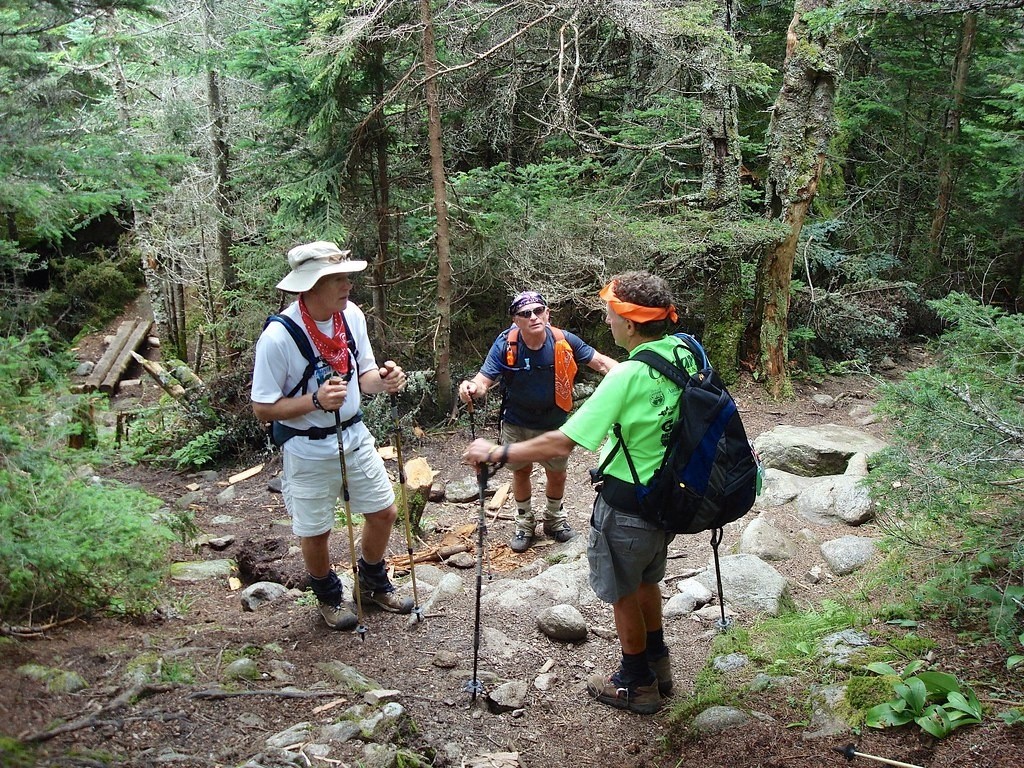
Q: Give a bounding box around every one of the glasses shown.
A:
[513,306,545,318]
[299,250,353,265]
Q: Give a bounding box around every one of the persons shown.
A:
[459,290,621,552]
[252,241,415,629]
[463,269,716,714]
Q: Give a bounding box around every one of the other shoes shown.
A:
[650,657,673,690]
[588,675,662,714]
[315,597,359,630]
[542,522,577,543]
[511,527,537,552]
[352,574,415,613]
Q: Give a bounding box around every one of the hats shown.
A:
[275,240,367,296]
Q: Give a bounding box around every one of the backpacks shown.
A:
[589,334,764,535]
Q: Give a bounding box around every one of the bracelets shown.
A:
[312,390,334,413]
[488,444,501,463]
